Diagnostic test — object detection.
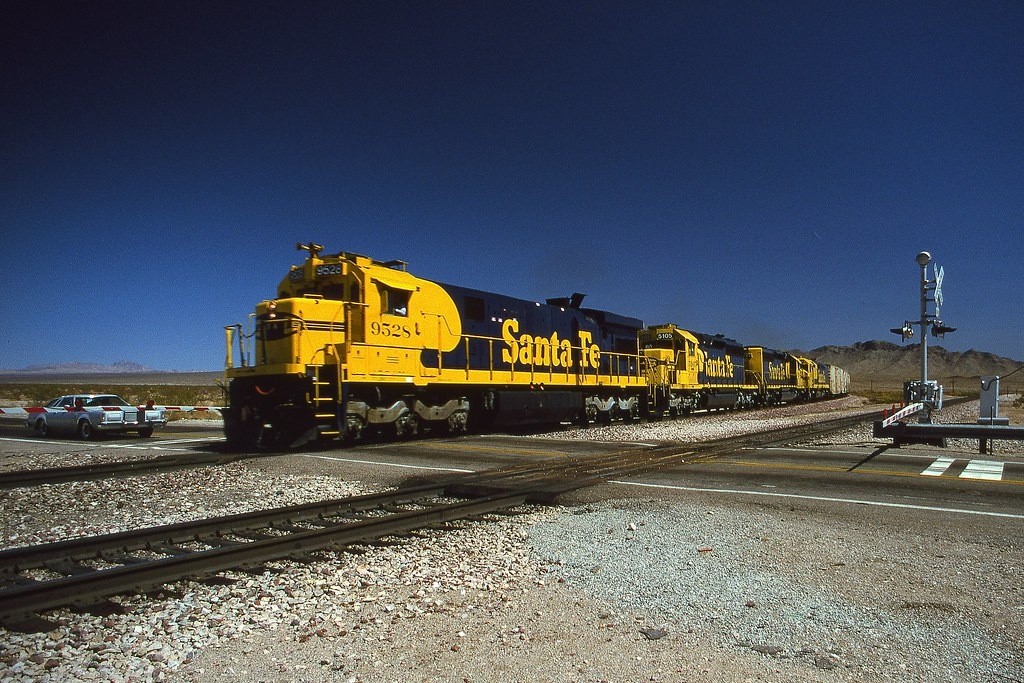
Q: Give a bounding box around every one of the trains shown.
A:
[220,243,852,454]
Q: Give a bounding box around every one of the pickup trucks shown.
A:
[27,394,165,442]
